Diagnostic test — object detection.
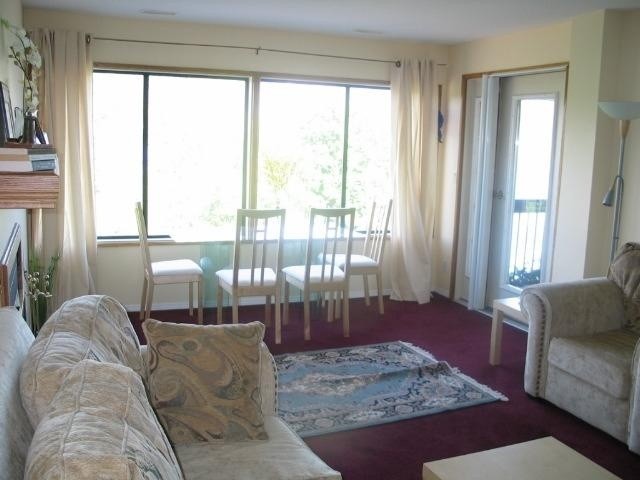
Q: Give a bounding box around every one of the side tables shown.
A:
[490,297,528,366]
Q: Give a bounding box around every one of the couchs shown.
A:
[0,307,342,480]
[519,277,640,455]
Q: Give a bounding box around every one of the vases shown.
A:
[24,117,37,144]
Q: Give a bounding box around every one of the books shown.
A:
[0,143,58,173]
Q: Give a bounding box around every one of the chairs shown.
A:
[134,200,395,344]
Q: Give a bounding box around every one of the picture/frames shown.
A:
[1,222,25,317]
[0,82,15,141]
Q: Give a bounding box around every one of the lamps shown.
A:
[597,102,640,264]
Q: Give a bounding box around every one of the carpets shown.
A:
[274,341,509,439]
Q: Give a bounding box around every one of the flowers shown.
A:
[0,17,42,117]
